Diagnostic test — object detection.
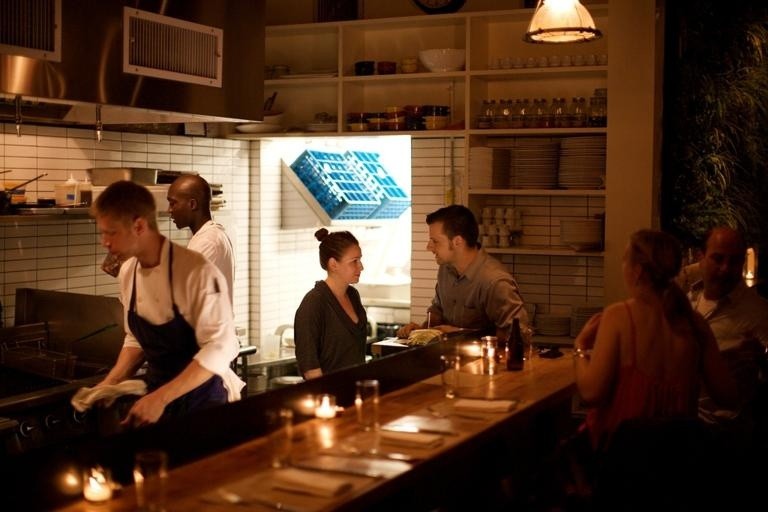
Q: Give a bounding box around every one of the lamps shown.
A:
[523,0,602,45]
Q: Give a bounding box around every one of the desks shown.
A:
[52,352,605,509]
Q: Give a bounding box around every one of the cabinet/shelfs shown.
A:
[222,1,609,344]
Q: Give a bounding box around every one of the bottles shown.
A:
[479,87,607,128]
[5,186,26,209]
[270,63,288,80]
[504,317,526,370]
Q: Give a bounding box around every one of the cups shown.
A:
[473,206,522,246]
[354,380,380,427]
[132,447,168,510]
[489,53,609,70]
[55,183,81,206]
[438,354,462,398]
[479,335,499,377]
[519,327,534,360]
[255,408,293,468]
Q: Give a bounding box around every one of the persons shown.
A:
[395,204,536,341]
[293,226,370,387]
[563,228,702,458]
[671,223,768,432]
[165,173,235,317]
[77,181,246,430]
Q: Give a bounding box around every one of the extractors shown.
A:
[0,0,265,131]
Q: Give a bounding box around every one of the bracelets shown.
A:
[571,343,591,360]
[459,326,465,331]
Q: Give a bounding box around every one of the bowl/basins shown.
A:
[346,104,449,131]
[86,166,160,188]
[265,114,281,123]
[417,47,465,73]
[353,56,417,75]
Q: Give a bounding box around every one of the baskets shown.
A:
[290,149,411,220]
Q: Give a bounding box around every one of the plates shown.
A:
[303,122,337,132]
[534,304,603,339]
[557,217,603,249]
[468,137,607,189]
[234,124,280,133]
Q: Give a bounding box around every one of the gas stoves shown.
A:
[0,361,93,457]
